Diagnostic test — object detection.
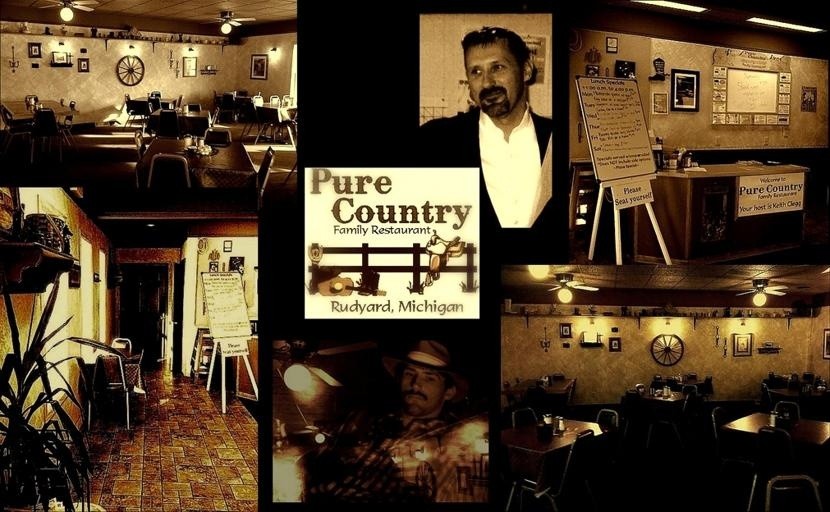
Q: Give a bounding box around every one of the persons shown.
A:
[419,26,556,284]
[301,339,491,501]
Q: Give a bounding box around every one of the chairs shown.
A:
[68,328,147,441]
[500,373,830,512]
[1,83,298,198]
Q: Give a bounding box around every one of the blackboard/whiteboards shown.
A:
[201,271,252,339]
[575,75,657,183]
[726,67,779,115]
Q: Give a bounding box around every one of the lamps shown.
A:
[750,291,768,307]
[219,22,233,34]
[58,7,73,21]
[557,288,573,305]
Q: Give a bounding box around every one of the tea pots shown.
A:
[183,134,193,148]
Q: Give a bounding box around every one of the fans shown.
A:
[734,277,791,300]
[39,0,99,13]
[199,7,257,26]
[534,272,600,292]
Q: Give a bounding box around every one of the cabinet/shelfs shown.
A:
[623,161,810,261]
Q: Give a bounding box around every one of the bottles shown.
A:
[183,102,188,113]
[663,386,671,397]
[555,416,565,432]
[199,139,204,148]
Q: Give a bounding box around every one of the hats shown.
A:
[382,338,468,405]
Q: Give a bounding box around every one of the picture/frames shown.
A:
[182,56,198,78]
[823,329,830,360]
[28,42,43,59]
[250,53,268,81]
[77,57,89,73]
[670,68,700,113]
[609,337,622,352]
[51,52,68,64]
[558,322,573,339]
[733,333,752,357]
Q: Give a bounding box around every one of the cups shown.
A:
[543,414,553,424]
